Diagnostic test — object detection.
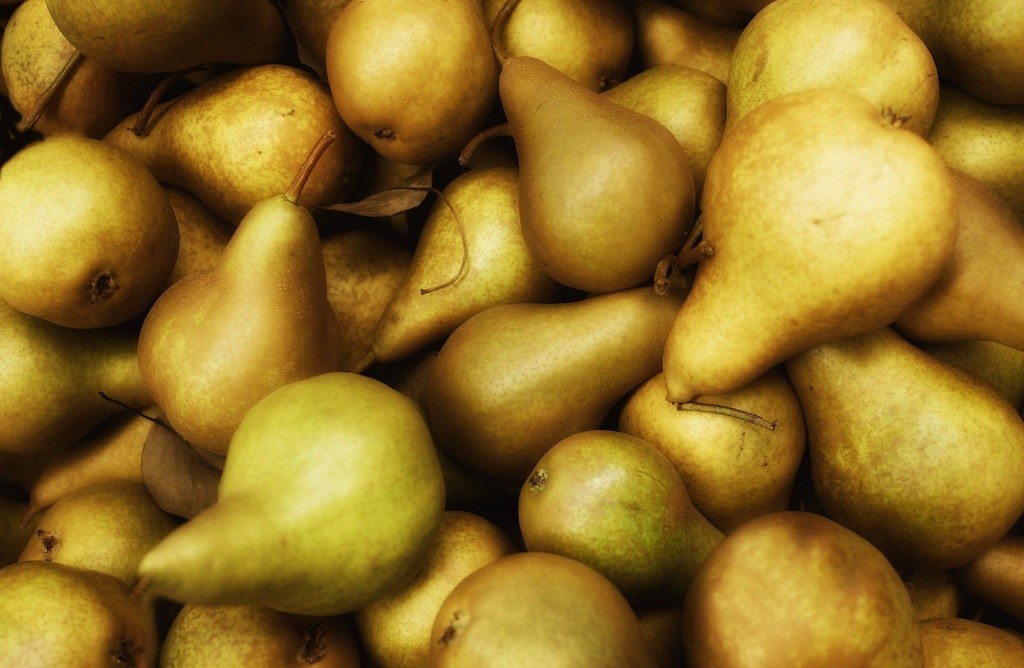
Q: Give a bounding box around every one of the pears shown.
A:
[0,0,1023,668]
